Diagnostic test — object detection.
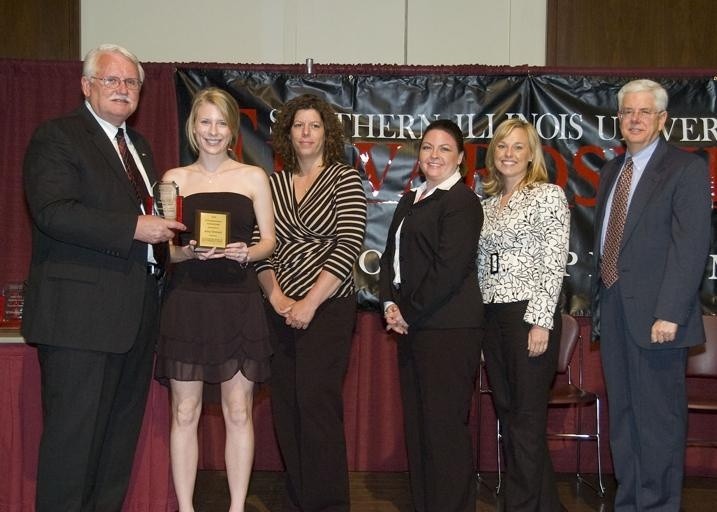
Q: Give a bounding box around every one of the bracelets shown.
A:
[240,248,248,269]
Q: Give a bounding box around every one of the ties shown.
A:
[600,158,633,288]
[116,129,168,264]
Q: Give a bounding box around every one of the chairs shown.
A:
[474,314,610,511]
[680,312,716,448]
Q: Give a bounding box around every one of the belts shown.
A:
[147,265,164,281]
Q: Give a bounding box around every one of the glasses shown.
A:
[90,75,142,90]
[618,108,658,118]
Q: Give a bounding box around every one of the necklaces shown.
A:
[196,164,220,185]
[299,171,316,193]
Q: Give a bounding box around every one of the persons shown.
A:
[155,88,278,512]
[589,79,712,511]
[477,118,572,512]
[19,43,186,512]
[378,120,483,512]
[250,92,368,511]
